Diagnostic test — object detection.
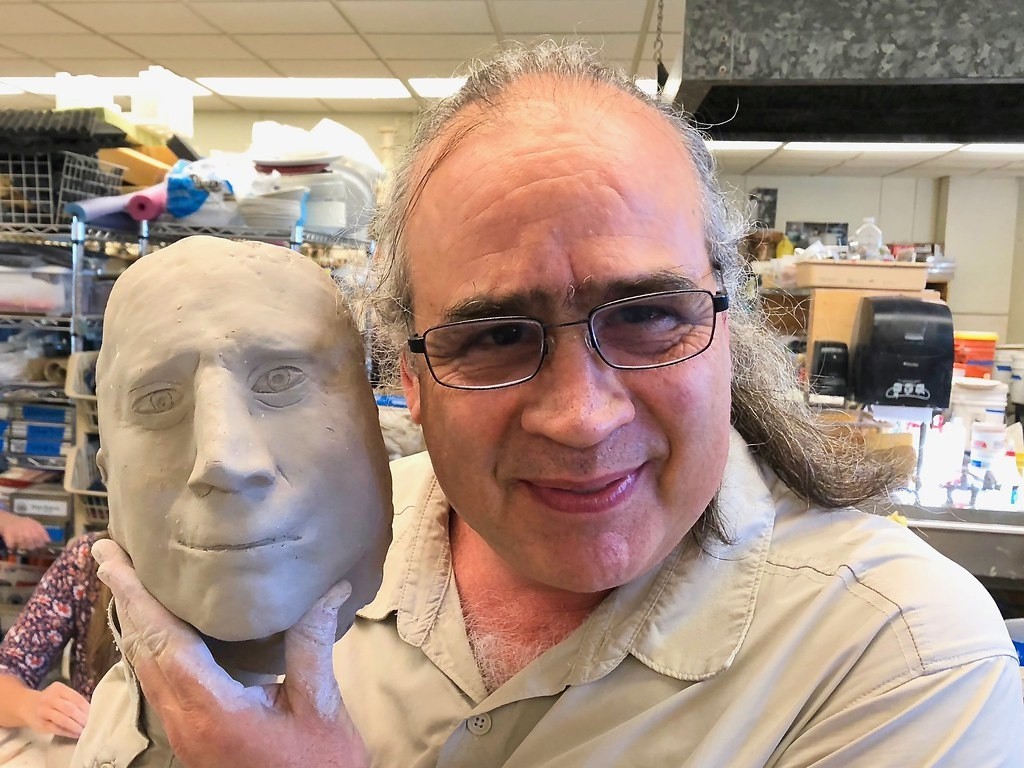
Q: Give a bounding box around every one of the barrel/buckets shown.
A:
[941,332,1024,455]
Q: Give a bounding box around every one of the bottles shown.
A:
[787,225,800,242]
[856,217,882,260]
[776,236,793,260]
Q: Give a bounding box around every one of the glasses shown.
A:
[407,289,730,393]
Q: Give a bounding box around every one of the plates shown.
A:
[230,149,376,235]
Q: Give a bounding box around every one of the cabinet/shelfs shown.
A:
[0,217,376,679]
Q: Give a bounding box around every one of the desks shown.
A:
[758,286,939,395]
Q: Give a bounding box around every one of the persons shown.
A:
[0,507,51,549]
[0,532,123,739]
[72,42,1024,768]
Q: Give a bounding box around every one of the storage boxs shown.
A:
[9,484,73,523]
[35,523,70,548]
[794,260,932,290]
